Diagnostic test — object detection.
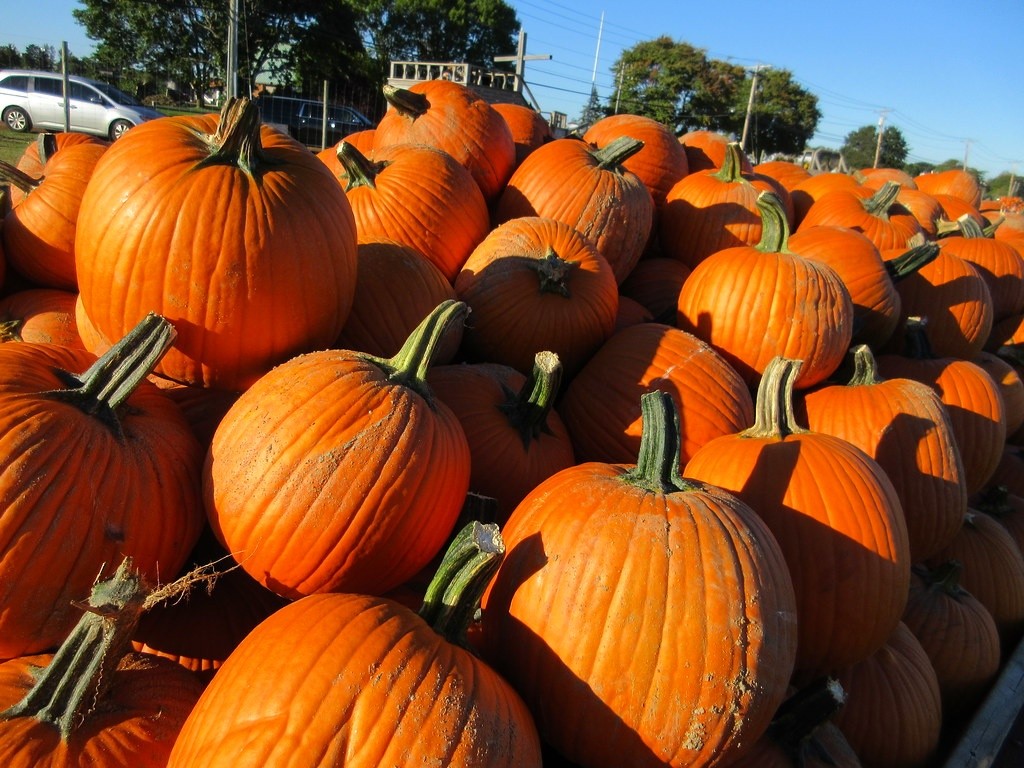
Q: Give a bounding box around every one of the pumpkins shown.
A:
[0,79,1024,768]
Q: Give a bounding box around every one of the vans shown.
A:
[254,95,375,155]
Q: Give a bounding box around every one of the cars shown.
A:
[0,68,168,141]
